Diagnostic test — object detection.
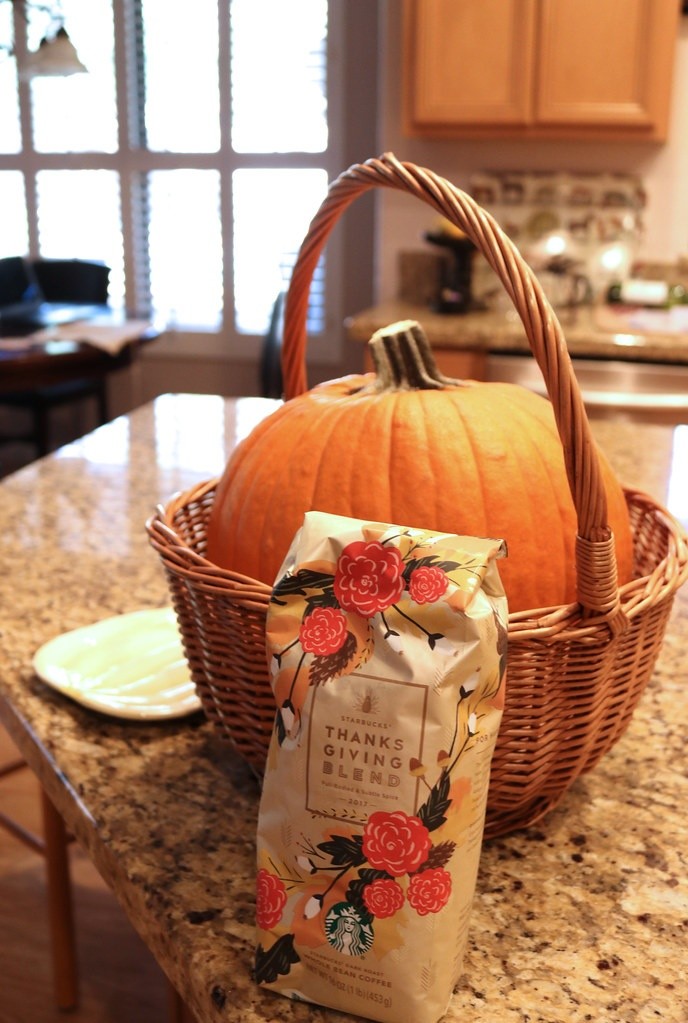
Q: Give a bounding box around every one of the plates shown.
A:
[31,608,202,721]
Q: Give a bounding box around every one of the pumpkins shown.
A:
[204,320,636,614]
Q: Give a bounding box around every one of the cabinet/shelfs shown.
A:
[366,345,479,380]
[396,0,684,147]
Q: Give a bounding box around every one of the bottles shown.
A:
[609,279,688,309]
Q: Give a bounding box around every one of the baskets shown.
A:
[143,153,688,840]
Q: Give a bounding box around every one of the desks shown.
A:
[1,390,688,1023]
[0,324,165,476]
